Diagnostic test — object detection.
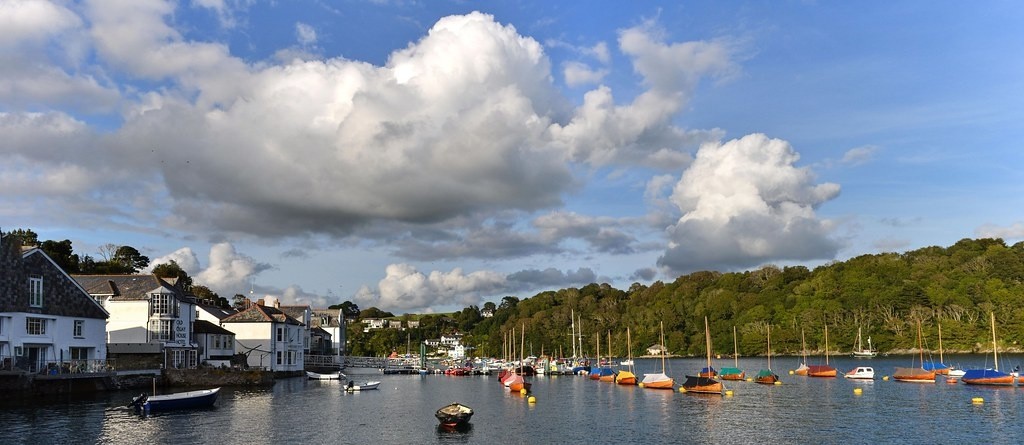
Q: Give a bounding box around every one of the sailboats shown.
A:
[598,329,616,383]
[532,308,591,375]
[892,319,936,383]
[719,326,745,380]
[589,331,602,380]
[795,327,810,376]
[752,323,779,384]
[678,315,723,394]
[850,328,877,358]
[498,322,531,391]
[960,311,1014,385]
[639,321,674,389]
[615,324,639,385]
[807,326,837,377]
[923,323,954,375]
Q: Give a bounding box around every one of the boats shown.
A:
[305,371,346,379]
[949,369,966,376]
[419,365,493,376]
[435,402,474,427]
[131,377,220,412]
[620,360,635,366]
[844,366,875,379]
[343,381,380,391]
